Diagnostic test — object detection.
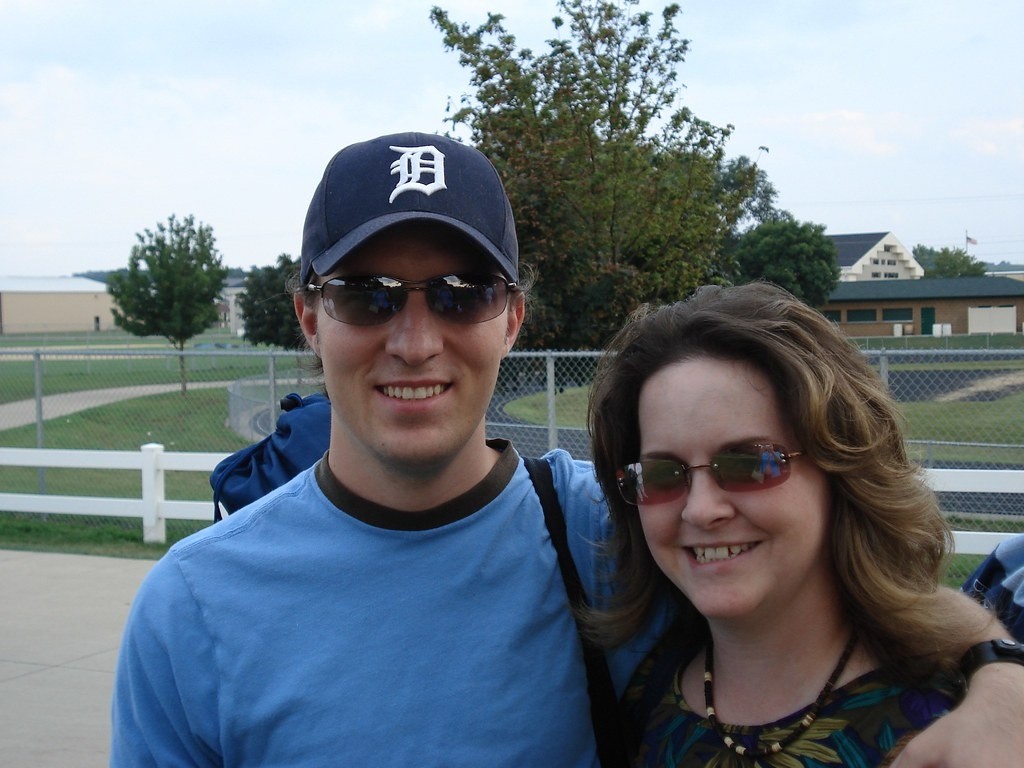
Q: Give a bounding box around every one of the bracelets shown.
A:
[957,639,1024,685]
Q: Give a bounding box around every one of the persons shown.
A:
[583,280,967,768]
[109,133,1024,767]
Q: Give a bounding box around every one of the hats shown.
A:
[301,132,519,287]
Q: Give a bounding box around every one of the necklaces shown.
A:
[699,609,859,763]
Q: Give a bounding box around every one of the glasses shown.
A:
[304,271,517,325]
[615,444,806,505]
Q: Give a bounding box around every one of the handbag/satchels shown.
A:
[751,463,765,484]
